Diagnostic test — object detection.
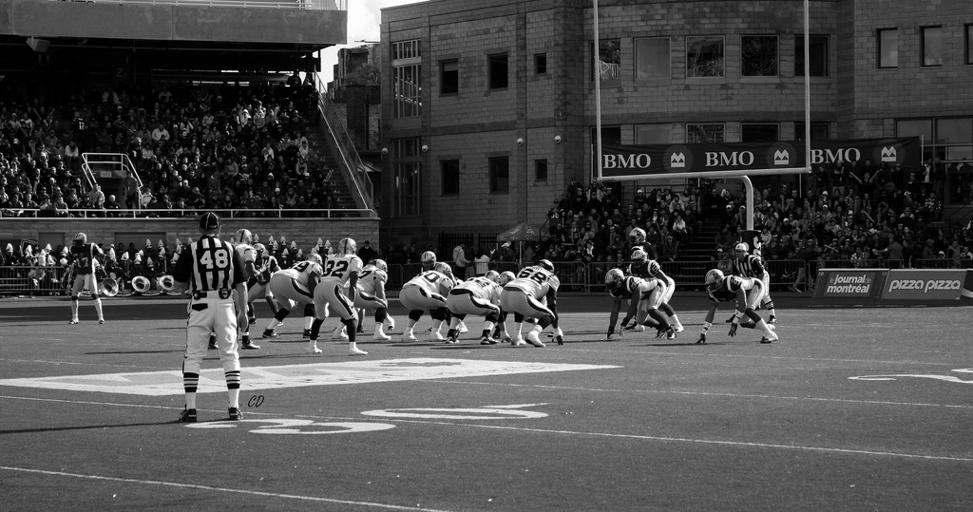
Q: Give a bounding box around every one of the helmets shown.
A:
[605,268,625,289]
[628,227,648,268]
[305,254,322,265]
[235,229,266,253]
[72,232,87,246]
[539,258,555,273]
[373,259,388,272]
[485,271,515,286]
[704,269,724,293]
[735,243,746,251]
[200,212,220,231]
[420,251,452,276]
[338,237,356,253]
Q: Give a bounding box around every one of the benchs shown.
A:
[513,168,971,293]
[0,69,366,294]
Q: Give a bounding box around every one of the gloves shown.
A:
[552,327,564,345]
[697,333,705,344]
[728,323,737,337]
[601,326,626,341]
[492,325,512,343]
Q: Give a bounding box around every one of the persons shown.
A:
[0,64,973,424]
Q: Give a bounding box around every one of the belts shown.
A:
[199,293,226,297]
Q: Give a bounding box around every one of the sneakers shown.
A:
[69,319,79,324]
[761,315,778,343]
[625,318,645,331]
[241,340,260,349]
[373,320,395,339]
[99,320,104,324]
[656,324,684,339]
[209,340,218,349]
[249,318,256,324]
[179,409,197,422]
[332,328,349,340]
[348,348,368,356]
[480,335,498,347]
[424,322,468,344]
[229,407,244,420]
[402,326,418,343]
[303,328,320,337]
[308,344,322,353]
[263,322,283,337]
[511,333,526,345]
[525,329,545,347]
